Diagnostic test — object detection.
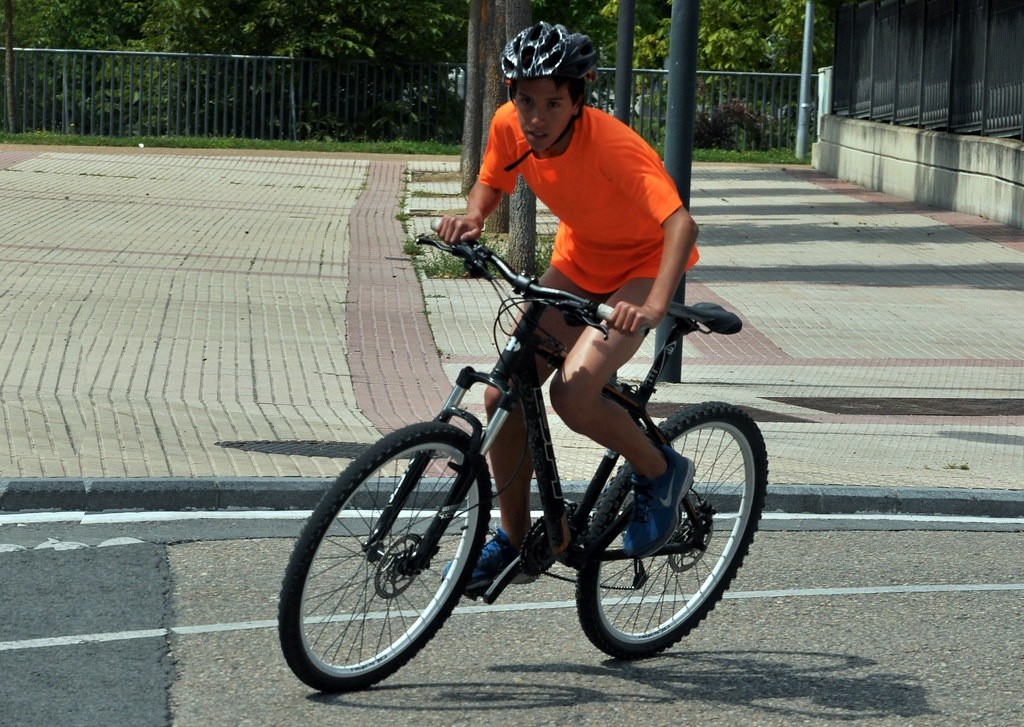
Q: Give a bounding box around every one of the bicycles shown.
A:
[277,216,770,693]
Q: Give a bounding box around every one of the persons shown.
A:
[440,21,699,593]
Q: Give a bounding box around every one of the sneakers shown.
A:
[623,445,695,559]
[441,527,540,590]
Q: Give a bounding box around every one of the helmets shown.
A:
[499,21,596,79]
